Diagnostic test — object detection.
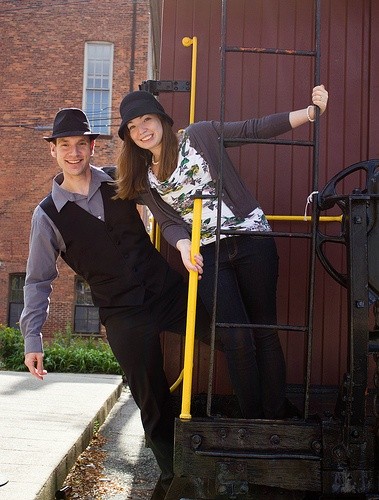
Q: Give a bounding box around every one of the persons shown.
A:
[115,83,329,423]
[17,105,273,496]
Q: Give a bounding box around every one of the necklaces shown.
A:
[151,152,160,165]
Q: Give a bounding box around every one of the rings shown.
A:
[319,94,323,101]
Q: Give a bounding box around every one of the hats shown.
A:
[44,108,101,143]
[118,90,173,140]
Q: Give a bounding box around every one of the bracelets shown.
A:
[306,105,316,123]
